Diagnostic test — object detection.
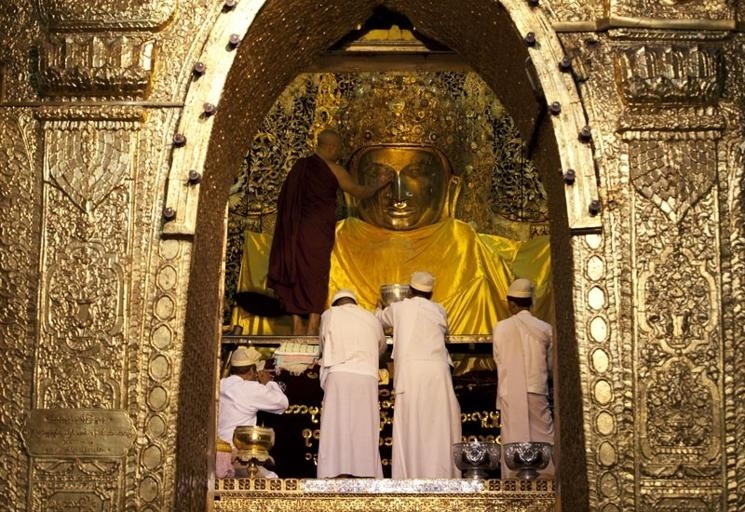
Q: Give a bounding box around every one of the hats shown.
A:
[409,272,434,293]
[332,289,357,305]
[231,348,262,367]
[507,279,534,298]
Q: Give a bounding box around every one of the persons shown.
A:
[493,279,557,482]
[215,345,290,478]
[268,129,395,336]
[231,71,556,336]
[373,272,461,479]
[315,290,388,479]
